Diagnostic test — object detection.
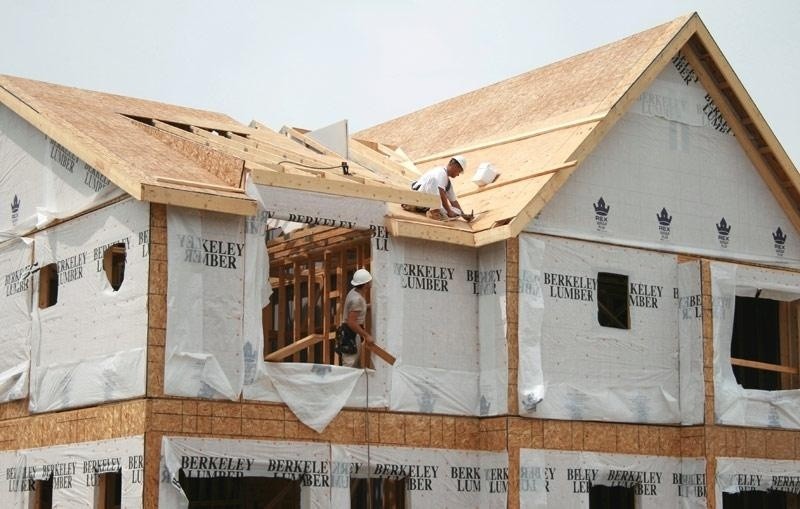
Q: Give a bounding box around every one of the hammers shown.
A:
[457,209,474,223]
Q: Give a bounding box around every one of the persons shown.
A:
[335,268,375,368]
[409,155,471,222]
